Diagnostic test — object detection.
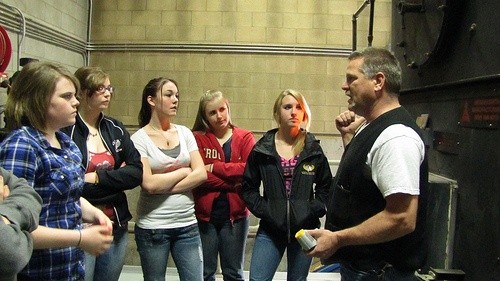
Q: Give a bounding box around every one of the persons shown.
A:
[130,77,208,281]
[73,66,144,281]
[0,167,43,281]
[194,91,260,281]
[302,47,430,281]
[0,60,114,281]
[243,89,334,281]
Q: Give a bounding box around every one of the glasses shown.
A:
[97,85,113,93]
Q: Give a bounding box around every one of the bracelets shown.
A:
[76,230,84,250]
[95,170,99,186]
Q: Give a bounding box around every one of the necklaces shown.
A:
[149,122,170,146]
[90,132,98,138]
